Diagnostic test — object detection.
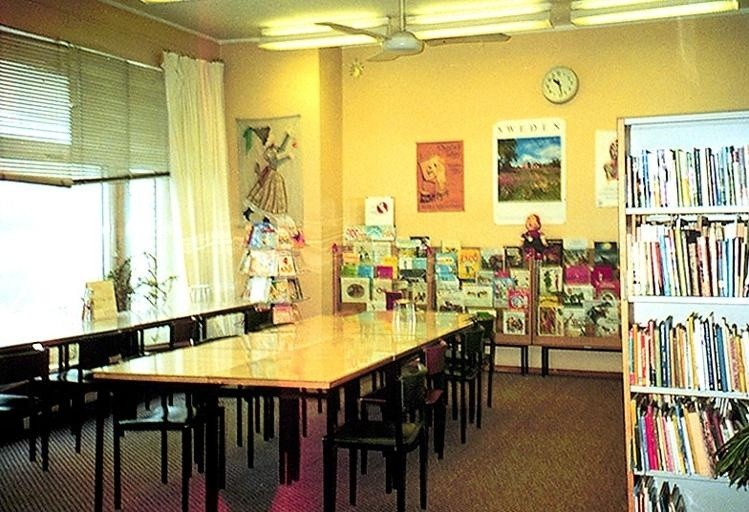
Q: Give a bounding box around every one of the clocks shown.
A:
[540,66,580,105]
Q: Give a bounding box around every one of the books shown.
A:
[86,279,119,323]
[634,476,687,512]
[628,312,749,393]
[626,146,749,208]
[241,222,307,323]
[627,216,749,297]
[631,394,749,479]
[334,237,619,342]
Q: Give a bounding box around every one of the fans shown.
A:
[313,0,514,64]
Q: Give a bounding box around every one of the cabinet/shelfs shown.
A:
[612,111,747,511]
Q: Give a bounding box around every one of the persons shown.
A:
[518,213,550,262]
